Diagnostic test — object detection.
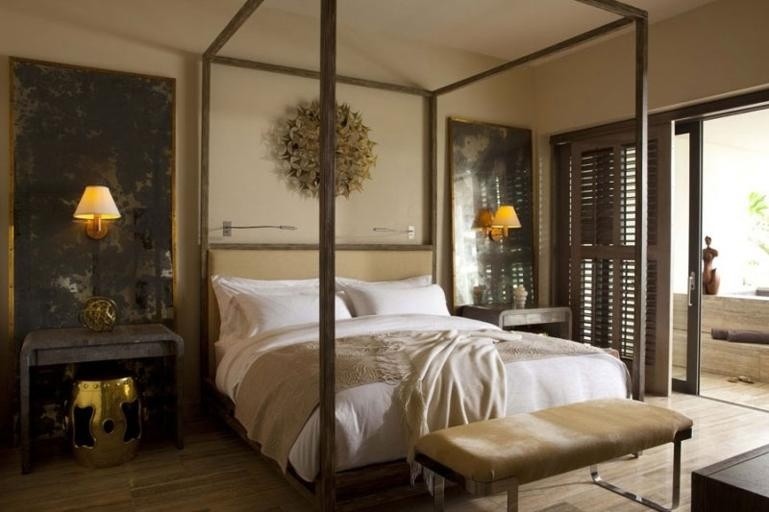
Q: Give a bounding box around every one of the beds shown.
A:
[202,0,650,510]
[74,186,122,333]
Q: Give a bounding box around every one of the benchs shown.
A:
[411,397,695,512]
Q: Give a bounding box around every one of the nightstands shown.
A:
[460,303,573,341]
[19,325,184,473]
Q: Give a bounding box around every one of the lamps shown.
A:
[471,207,494,238]
[487,204,522,243]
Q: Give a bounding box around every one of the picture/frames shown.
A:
[8,52,184,454]
[448,117,541,317]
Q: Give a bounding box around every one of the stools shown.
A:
[67,373,144,470]
